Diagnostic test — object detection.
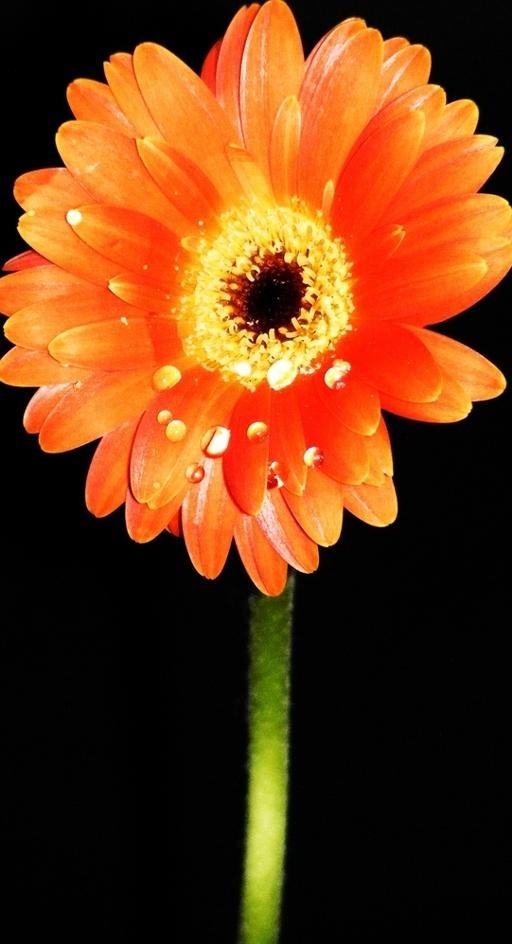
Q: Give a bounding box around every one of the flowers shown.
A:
[0,0,512,944]
